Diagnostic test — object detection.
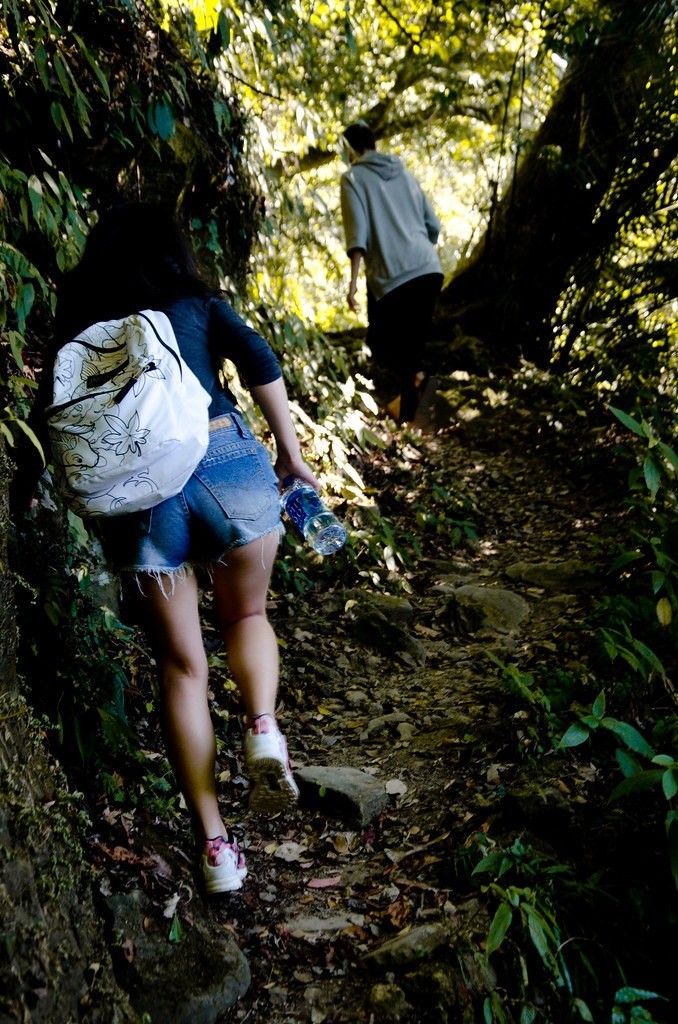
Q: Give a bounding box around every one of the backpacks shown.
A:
[45,310,212,517]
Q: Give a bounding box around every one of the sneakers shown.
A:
[201,834,247,894]
[243,713,298,813]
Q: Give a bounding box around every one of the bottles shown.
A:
[279,473,347,554]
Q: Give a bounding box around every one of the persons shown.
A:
[341,121,445,433]
[10,204,323,892]
